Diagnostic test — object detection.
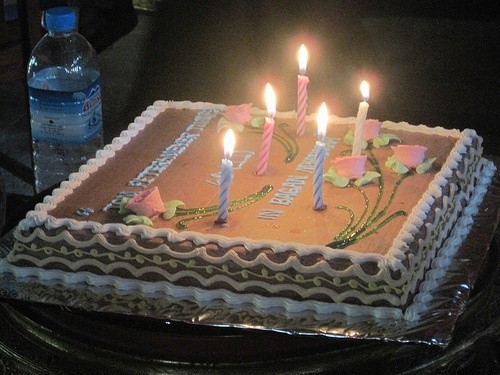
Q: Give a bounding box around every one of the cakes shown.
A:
[1,99,497,323]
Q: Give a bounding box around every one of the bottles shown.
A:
[24,8,106,194]
